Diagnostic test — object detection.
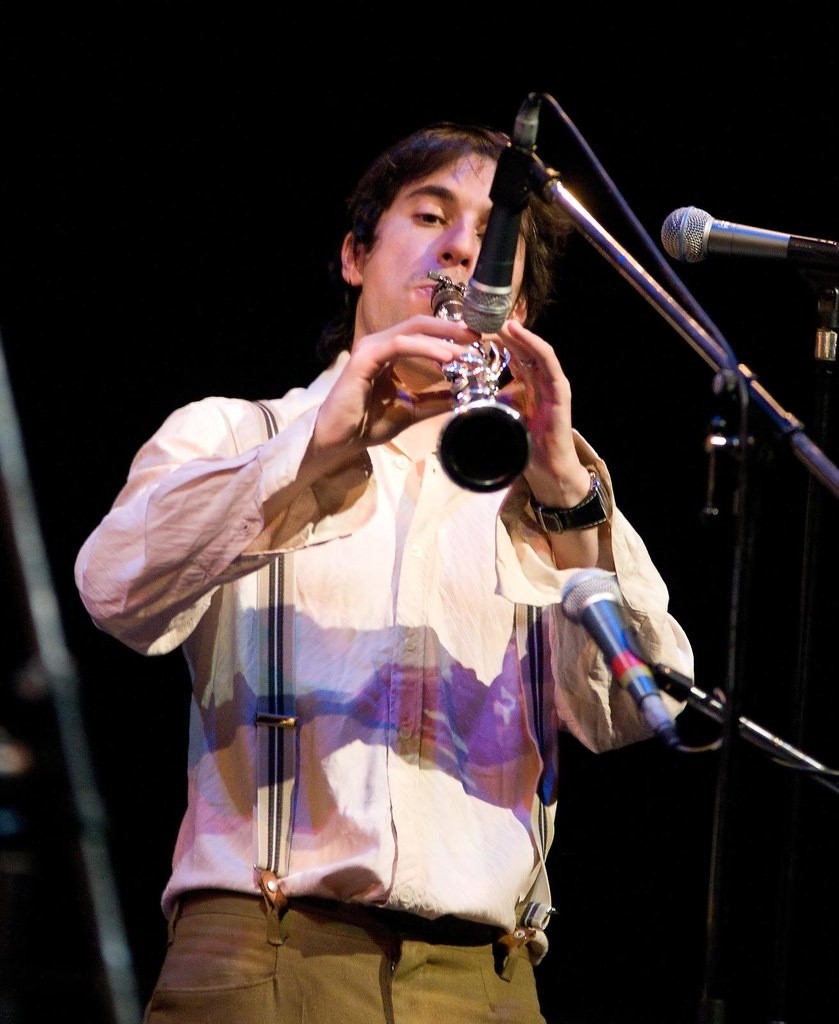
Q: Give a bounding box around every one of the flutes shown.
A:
[425,271,537,492]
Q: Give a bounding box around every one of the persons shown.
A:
[74,128,695,1024]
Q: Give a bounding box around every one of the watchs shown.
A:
[529,473,612,535]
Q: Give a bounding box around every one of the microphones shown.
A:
[561,568,682,751]
[662,207,838,269]
[462,94,539,335]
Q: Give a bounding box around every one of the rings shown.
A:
[525,361,535,368]
